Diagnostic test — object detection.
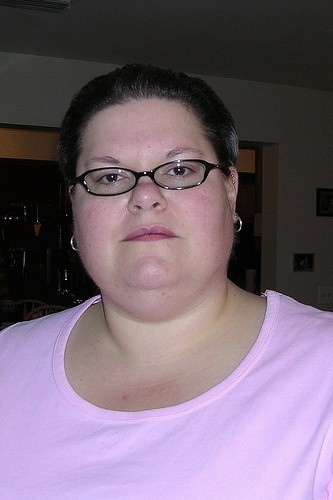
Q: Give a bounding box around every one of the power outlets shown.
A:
[317,286,333,305]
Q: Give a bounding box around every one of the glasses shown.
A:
[69,158,230,197]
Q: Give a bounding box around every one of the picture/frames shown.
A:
[315,187,332,218]
[290,253,317,272]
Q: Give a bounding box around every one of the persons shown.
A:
[1,60,332,500]
